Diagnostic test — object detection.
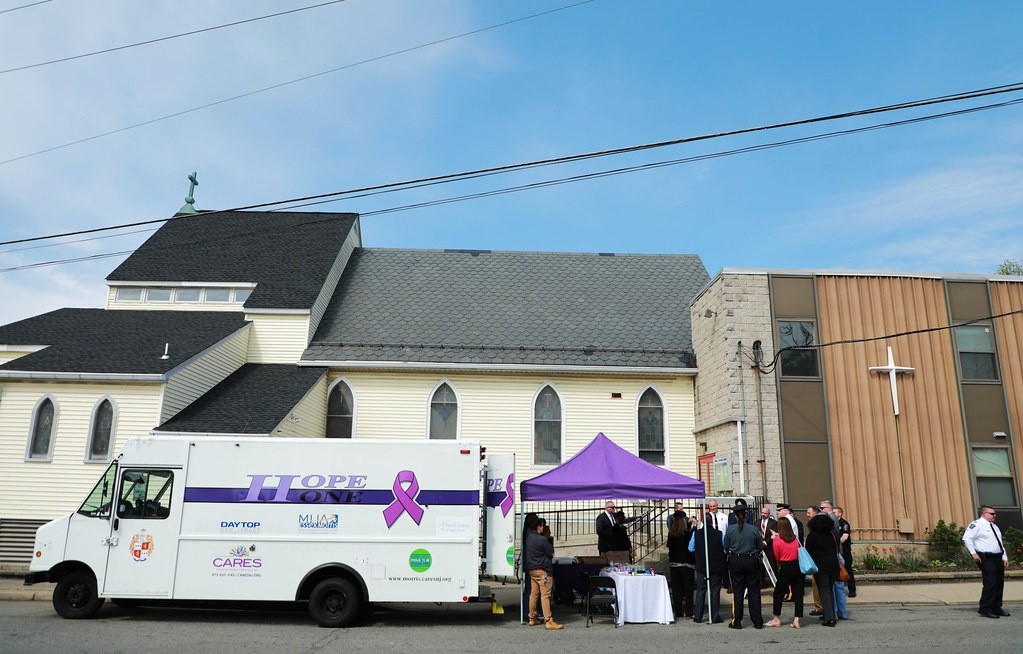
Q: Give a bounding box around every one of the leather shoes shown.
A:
[994,608,1010,616]
[978,610,1000,618]
[822,619,836,627]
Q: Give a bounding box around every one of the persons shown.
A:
[723,506,764,629]
[770,503,804,602]
[596,502,642,563]
[962,506,1011,619]
[669,500,728,618]
[806,500,857,627]
[755,508,778,588]
[728,499,753,526]
[523,513,563,630]
[764,517,803,628]
[688,513,726,623]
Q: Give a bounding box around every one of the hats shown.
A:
[776,503,790,511]
[673,510,686,520]
[733,506,747,512]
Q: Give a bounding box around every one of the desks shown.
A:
[599,571,674,627]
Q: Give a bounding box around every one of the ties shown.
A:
[990,524,1003,551]
[761,520,765,539]
[714,515,718,530]
[611,515,615,524]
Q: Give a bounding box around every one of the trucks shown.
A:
[20,427,521,628]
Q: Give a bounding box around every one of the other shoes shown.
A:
[849,590,856,598]
[729,623,742,628]
[810,611,823,615]
[694,618,701,623]
[791,621,800,628]
[713,618,724,623]
[763,619,781,626]
[753,623,762,629]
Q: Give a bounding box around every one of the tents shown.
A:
[520,432,713,625]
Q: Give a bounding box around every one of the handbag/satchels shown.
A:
[688,531,696,553]
[838,561,849,581]
[796,537,818,575]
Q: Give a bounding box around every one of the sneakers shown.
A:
[546,617,563,629]
[529,619,541,625]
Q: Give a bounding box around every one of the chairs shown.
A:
[572,557,619,628]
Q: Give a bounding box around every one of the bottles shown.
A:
[769,528,775,535]
[609,561,645,576]
[650,567,654,575]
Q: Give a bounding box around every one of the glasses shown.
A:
[541,525,545,527]
[985,512,995,515]
[821,507,827,510]
[608,506,615,509]
